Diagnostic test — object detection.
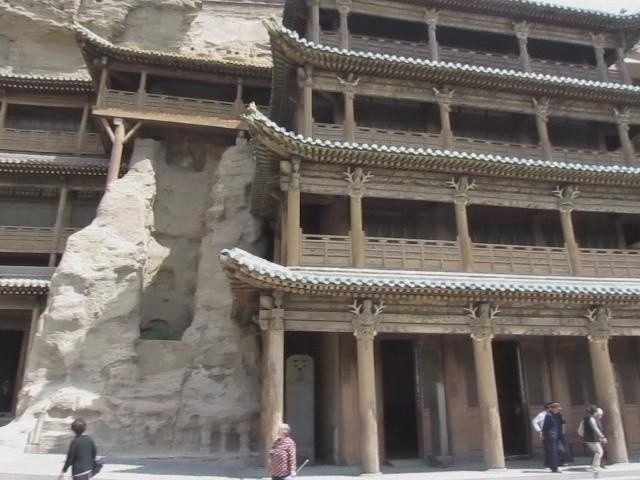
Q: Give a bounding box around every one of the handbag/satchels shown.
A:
[92,460,102,476]
[577,420,584,437]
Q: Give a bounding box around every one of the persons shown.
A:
[56,418,105,480]
[543,402,567,473]
[552,400,575,464]
[531,400,564,467]
[266,421,298,480]
[577,407,608,469]
[582,404,608,471]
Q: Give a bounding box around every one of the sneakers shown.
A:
[592,464,606,471]
[552,469,562,473]
